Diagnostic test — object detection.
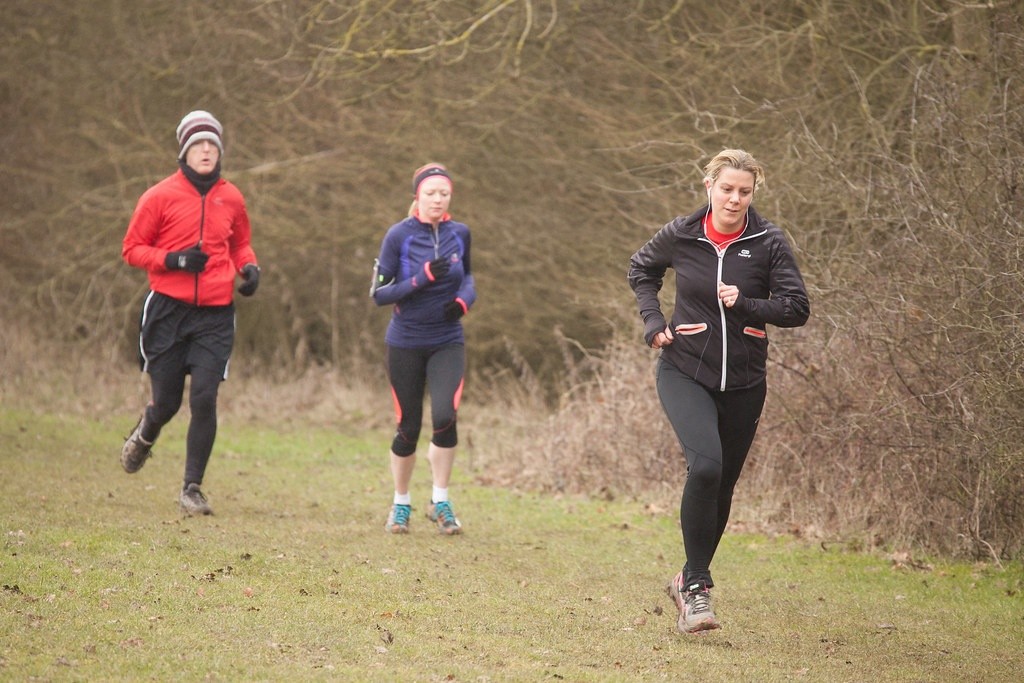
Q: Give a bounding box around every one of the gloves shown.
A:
[429,255,452,281]
[165,239,208,273]
[443,301,465,322]
[238,263,258,296]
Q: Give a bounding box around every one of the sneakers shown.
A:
[663,569,722,637]
[384,503,411,534]
[180,483,212,516]
[425,499,463,536]
[119,417,161,473]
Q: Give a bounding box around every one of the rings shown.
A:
[728,296,731,301]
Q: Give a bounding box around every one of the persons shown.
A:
[121,110,259,515]
[628,149,810,633]
[370,164,476,536]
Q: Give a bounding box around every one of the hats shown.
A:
[176,111,224,159]
[413,164,453,200]
